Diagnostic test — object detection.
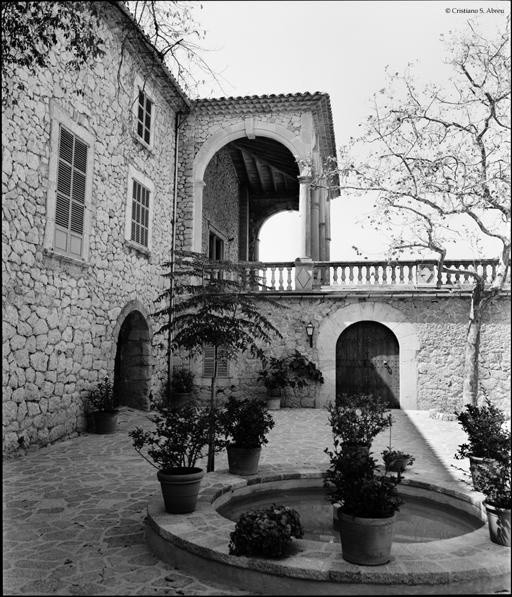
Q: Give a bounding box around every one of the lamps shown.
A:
[305,320,314,347]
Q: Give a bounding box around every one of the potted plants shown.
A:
[321,392,413,567]
[256,350,325,410]
[159,365,202,412]
[82,376,122,434]
[219,394,273,475]
[453,382,512,491]
[229,502,305,563]
[128,389,237,515]
[450,421,512,548]
